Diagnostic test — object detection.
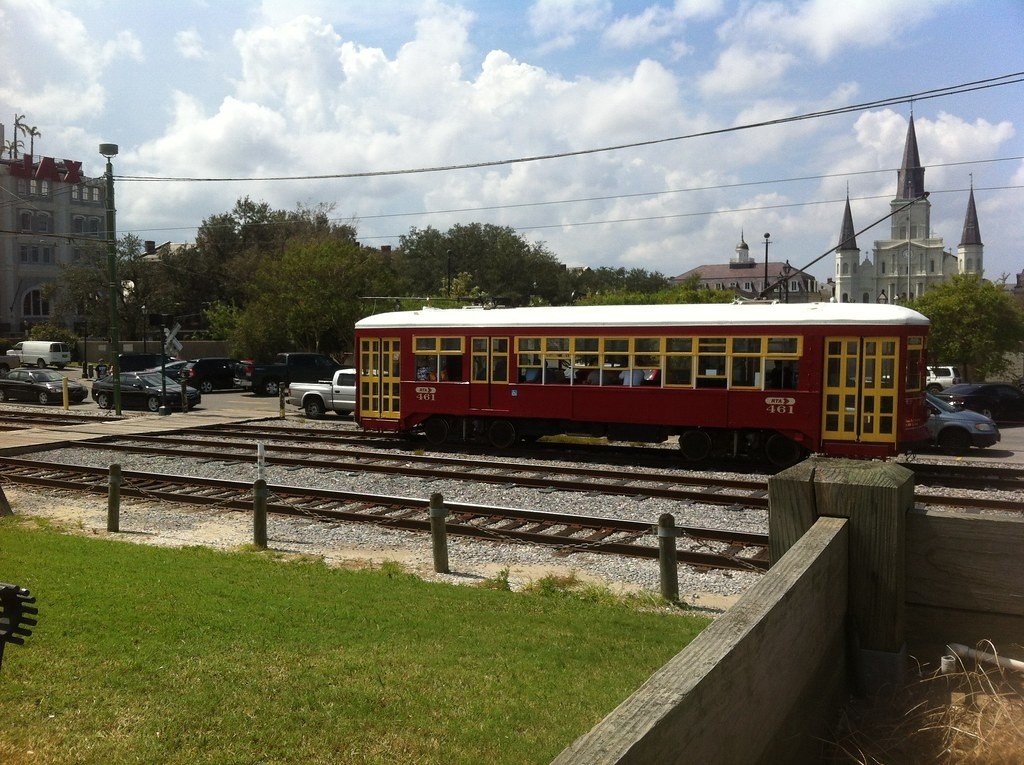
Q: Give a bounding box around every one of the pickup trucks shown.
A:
[0,356,20,378]
[232,352,354,397]
[284,367,390,420]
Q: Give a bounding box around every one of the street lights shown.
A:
[140,304,149,353]
[782,259,791,301]
[98,143,122,414]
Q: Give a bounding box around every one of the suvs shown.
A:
[110,352,174,372]
[926,365,962,396]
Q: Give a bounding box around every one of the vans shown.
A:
[6,340,72,369]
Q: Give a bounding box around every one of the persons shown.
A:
[95,358,108,378]
[417,356,644,386]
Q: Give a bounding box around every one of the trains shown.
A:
[355,301,934,468]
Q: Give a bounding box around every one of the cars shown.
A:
[91,370,202,412]
[0,367,89,405]
[176,357,245,394]
[934,382,1023,422]
[924,389,1003,454]
[149,360,188,380]
